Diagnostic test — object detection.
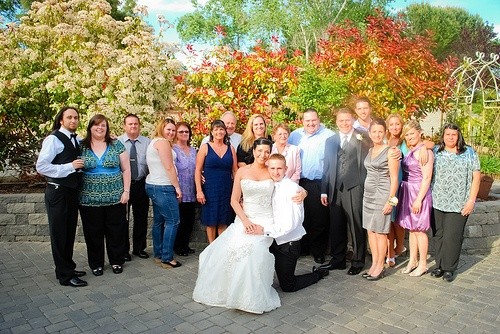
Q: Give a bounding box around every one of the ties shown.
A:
[71,134,79,152]
[342,135,348,176]
[129,139,139,180]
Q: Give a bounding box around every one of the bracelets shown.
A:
[124,190,129,193]
[388,197,398,206]
[468,199,476,204]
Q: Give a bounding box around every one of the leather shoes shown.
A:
[314,253,325,264]
[312,266,329,276]
[320,264,346,270]
[72,270,87,277]
[430,267,442,278]
[59,278,87,287]
[443,273,453,282]
[347,267,362,275]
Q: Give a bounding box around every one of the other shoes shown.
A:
[93,267,104,276]
[132,250,149,259]
[173,246,189,257]
[124,253,132,262]
[184,246,195,253]
[112,264,123,274]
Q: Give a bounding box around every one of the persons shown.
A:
[237,114,274,168]
[118,114,151,262]
[246,153,329,292]
[288,98,435,280]
[192,137,307,315]
[265,123,301,184]
[144,118,182,269]
[200,111,243,236]
[194,119,238,243]
[77,114,131,276]
[36,107,118,287]
[171,122,197,258]
[429,124,481,282]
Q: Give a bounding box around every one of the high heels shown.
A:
[363,267,386,280]
[386,247,407,268]
[154,255,162,263]
[402,261,419,274]
[409,268,429,277]
[162,259,182,268]
